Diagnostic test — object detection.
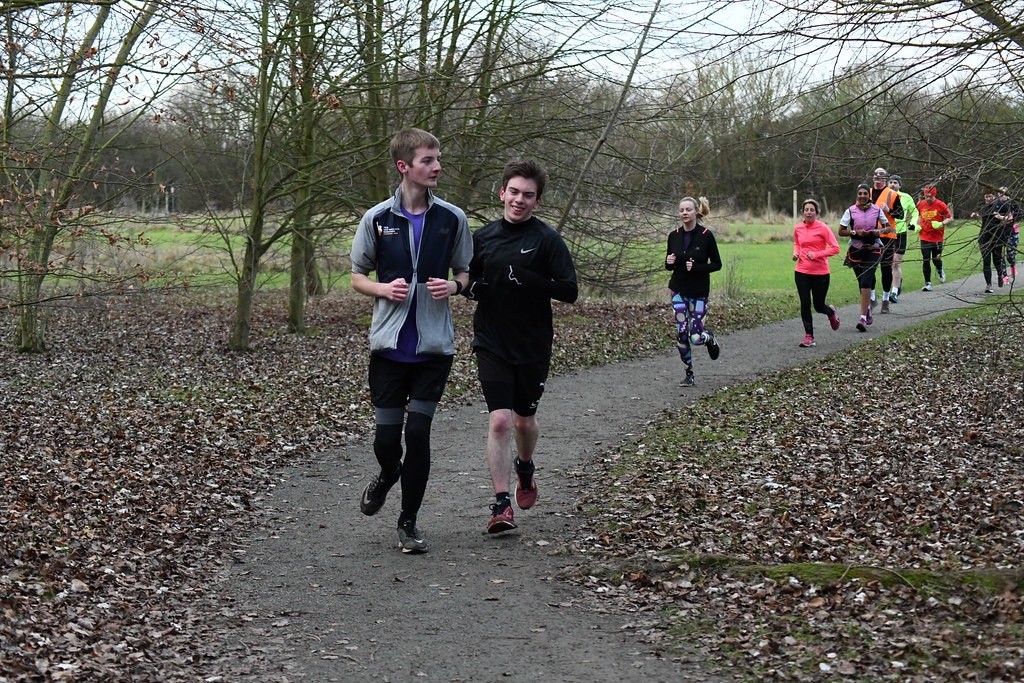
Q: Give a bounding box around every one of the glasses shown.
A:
[874,171,885,176]
[890,181,898,184]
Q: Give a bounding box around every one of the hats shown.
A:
[923,185,937,197]
[873,167,888,178]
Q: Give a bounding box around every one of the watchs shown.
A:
[852,230,856,236]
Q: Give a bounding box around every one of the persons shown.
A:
[664,197,722,387]
[915,185,952,291]
[792,199,839,347]
[868,167,904,314]
[350,127,473,552]
[840,183,891,330]
[971,186,1020,293]
[461,162,578,533]
[887,174,919,302]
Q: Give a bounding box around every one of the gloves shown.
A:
[915,225,922,232]
[503,264,538,288]
[465,277,490,300]
[931,220,944,229]
[908,224,915,231]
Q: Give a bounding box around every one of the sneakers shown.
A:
[513,454,537,509]
[396,520,428,553]
[828,305,840,330]
[856,267,1016,332]
[679,371,695,386]
[488,496,516,534]
[706,331,720,360]
[799,334,816,347]
[360,460,403,514]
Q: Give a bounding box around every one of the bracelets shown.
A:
[451,281,462,296]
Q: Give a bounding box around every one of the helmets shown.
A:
[858,191,868,195]
